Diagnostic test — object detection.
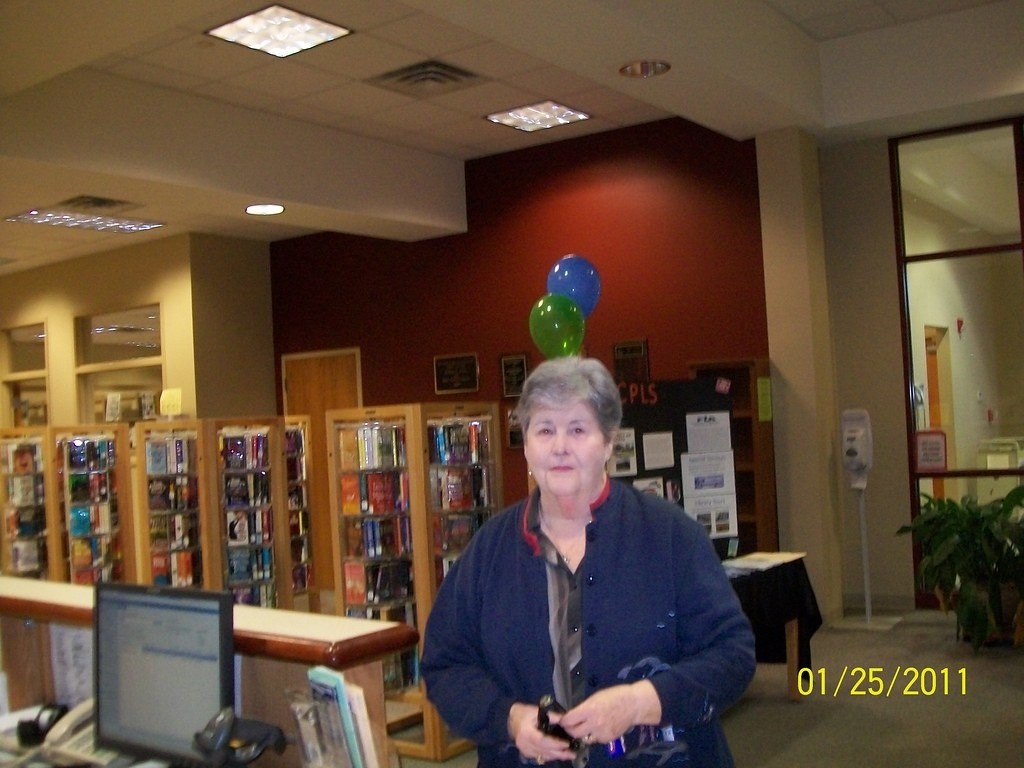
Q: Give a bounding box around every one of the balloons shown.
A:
[529,294,585,360]
[547,254,601,319]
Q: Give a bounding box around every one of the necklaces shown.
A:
[539,504,569,564]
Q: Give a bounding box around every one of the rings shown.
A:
[537,755,545,765]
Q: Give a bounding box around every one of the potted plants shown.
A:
[894,485,1024,656]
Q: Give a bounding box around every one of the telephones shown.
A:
[915,385,925,411]
[41,698,119,768]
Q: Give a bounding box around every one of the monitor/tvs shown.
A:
[91,581,242,768]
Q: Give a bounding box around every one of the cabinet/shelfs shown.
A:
[136,414,322,615]
[324,401,503,761]
[686,356,779,555]
[0,423,137,586]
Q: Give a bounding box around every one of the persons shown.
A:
[415,356,757,768]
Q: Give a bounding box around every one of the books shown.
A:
[336,422,491,689]
[6,441,49,570]
[290,666,382,768]
[145,438,203,587]
[217,430,313,610]
[57,438,123,587]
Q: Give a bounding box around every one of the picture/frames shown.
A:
[498,352,531,400]
[434,352,480,395]
[505,405,525,449]
[614,340,649,383]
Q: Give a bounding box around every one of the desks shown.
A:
[729,558,823,703]
